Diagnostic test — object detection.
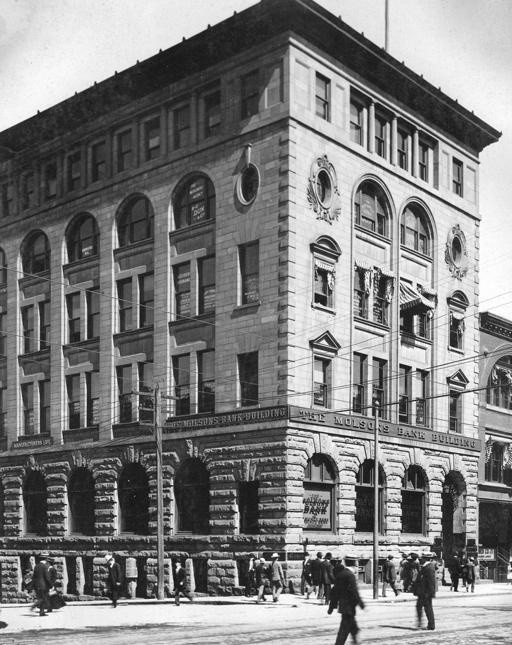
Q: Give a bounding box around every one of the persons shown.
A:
[246,544,477,645]
[174,558,194,606]
[108,558,121,607]
[47,559,57,613]
[30,557,54,615]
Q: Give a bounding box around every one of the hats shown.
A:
[422,551,437,558]
[343,554,360,561]
[270,552,281,558]
